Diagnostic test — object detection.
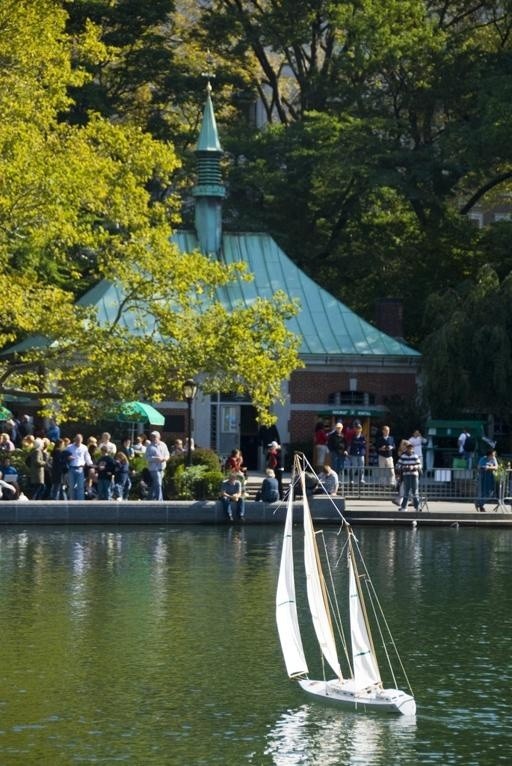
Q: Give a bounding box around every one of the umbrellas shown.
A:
[96,400,165,458]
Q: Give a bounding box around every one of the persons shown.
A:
[457,426,472,470]
[474,451,499,513]
[255,468,280,503]
[0,413,196,500]
[224,448,249,497]
[268,441,284,501]
[283,464,319,501]
[256,423,281,466]
[314,418,428,513]
[217,472,246,526]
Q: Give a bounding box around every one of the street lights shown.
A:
[179,376,200,468]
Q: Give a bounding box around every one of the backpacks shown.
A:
[462,432,477,452]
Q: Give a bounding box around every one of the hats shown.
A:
[335,422,344,428]
[268,441,279,448]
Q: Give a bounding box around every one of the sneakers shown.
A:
[225,514,246,522]
[398,507,423,512]
[475,501,486,513]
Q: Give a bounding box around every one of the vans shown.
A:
[423,417,492,470]
